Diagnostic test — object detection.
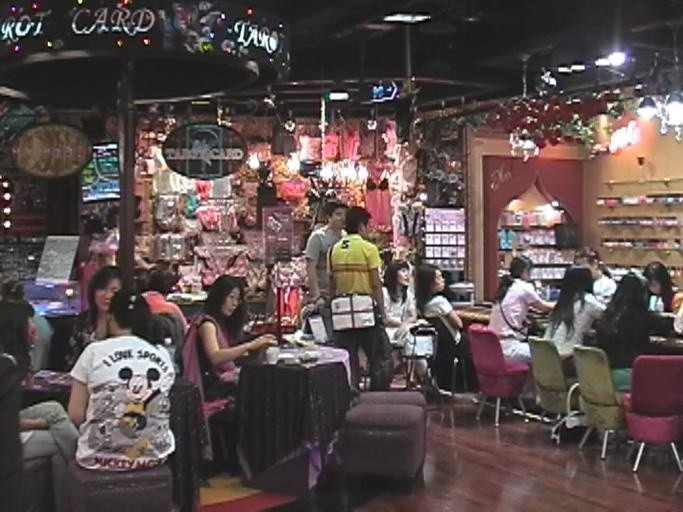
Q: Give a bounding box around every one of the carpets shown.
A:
[195,463,308,509]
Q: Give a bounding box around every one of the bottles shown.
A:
[657,296,664,312]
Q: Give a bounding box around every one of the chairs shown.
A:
[348,279,480,424]
[523,335,584,447]
[618,351,683,475]
[570,343,632,459]
[464,320,533,429]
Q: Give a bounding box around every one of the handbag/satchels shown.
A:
[553,207,581,247]
[303,314,332,343]
[551,415,602,444]
[331,295,376,331]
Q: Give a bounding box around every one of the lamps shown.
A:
[631,82,682,120]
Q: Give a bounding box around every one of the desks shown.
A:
[238,342,352,498]
[10,368,208,510]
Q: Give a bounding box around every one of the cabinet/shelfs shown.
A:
[594,176,683,254]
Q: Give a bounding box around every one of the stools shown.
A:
[48,445,173,510]
[13,421,57,511]
[340,403,424,495]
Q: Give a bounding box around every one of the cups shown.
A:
[266,346,334,364]
